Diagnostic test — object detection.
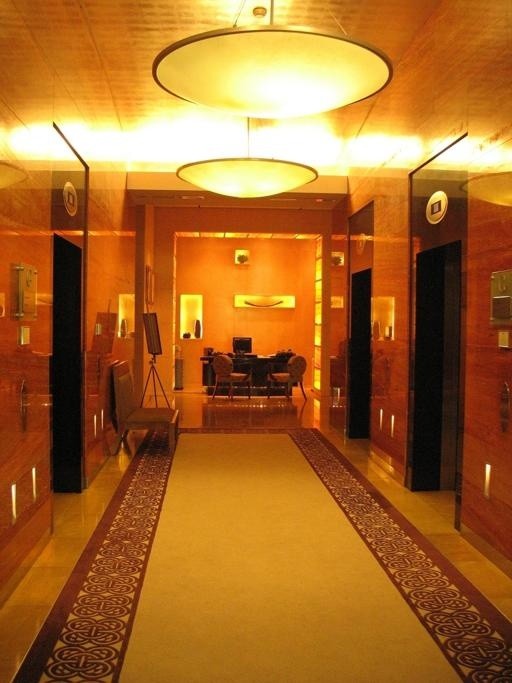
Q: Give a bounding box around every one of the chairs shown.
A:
[113,360,178,458]
[266,355,306,399]
[210,355,251,401]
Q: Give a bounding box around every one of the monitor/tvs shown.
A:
[233,337,252,355]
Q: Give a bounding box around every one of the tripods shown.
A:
[139,364,171,409]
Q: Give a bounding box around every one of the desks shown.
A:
[200,354,298,396]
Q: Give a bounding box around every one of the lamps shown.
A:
[175,118,319,199]
[152,0,392,120]
